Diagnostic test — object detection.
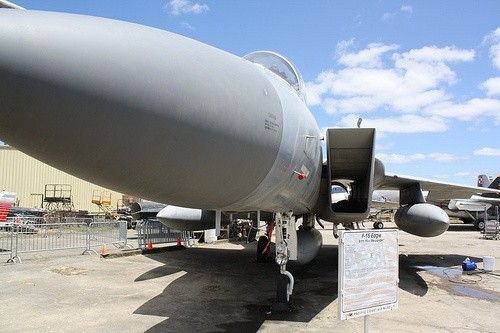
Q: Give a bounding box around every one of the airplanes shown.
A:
[0,2,500,312]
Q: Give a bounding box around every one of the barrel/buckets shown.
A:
[482,256,495,271]
